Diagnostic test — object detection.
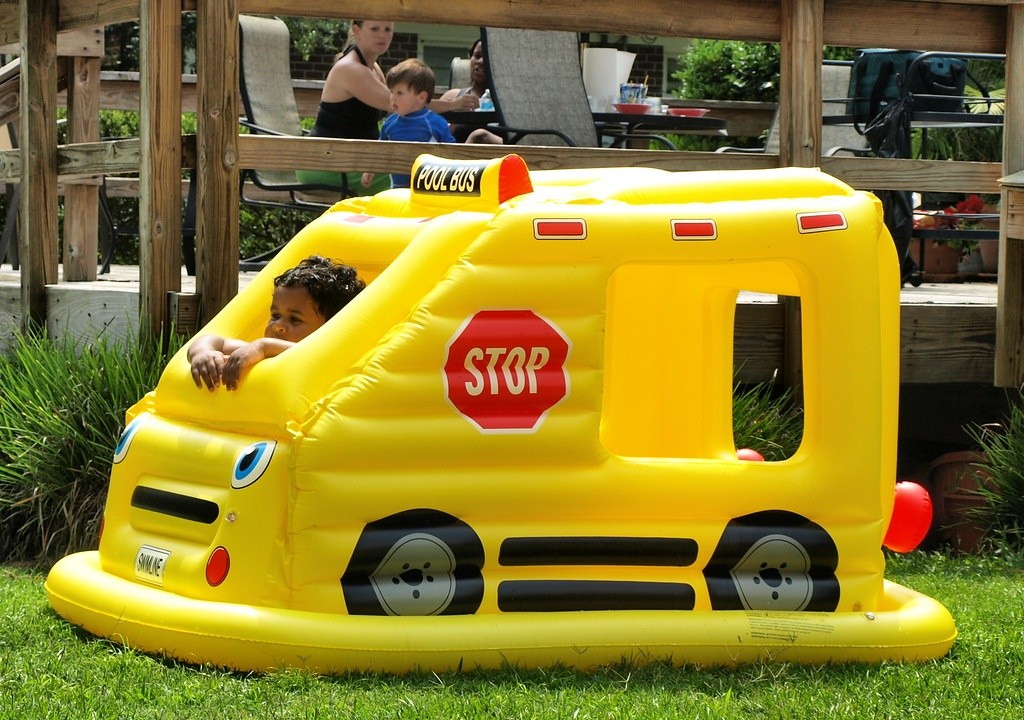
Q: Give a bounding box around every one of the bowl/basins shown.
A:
[612,103,652,114]
[667,108,711,118]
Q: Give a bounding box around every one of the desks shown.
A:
[437,109,730,152]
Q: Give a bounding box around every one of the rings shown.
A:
[471,102,475,108]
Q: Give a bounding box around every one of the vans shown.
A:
[42,152,961,666]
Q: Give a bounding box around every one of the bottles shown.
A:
[480,89,494,111]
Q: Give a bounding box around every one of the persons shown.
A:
[186,255,366,394]
[295,18,480,195]
[440,37,506,146]
[361,57,458,190]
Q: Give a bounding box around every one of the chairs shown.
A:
[236,14,358,273]
[478,24,677,150]
[713,60,875,157]
